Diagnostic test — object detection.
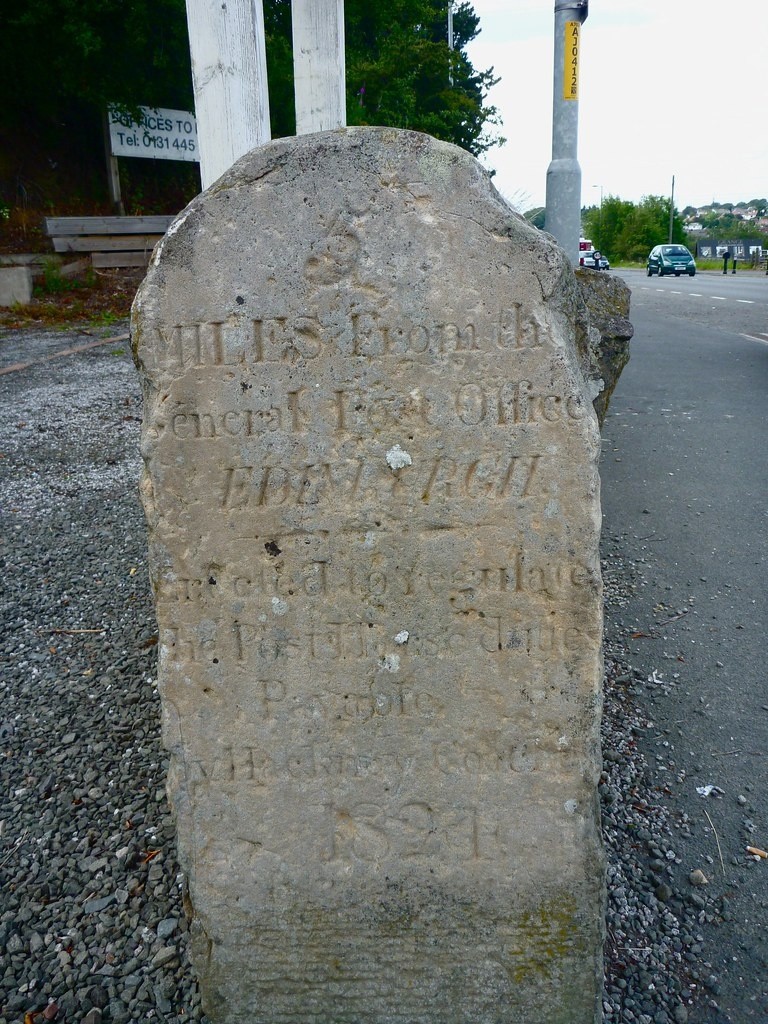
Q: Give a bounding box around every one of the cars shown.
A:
[578,251,601,267]
[600,255,610,271]
[646,244,696,277]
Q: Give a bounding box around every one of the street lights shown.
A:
[592,185,603,250]
